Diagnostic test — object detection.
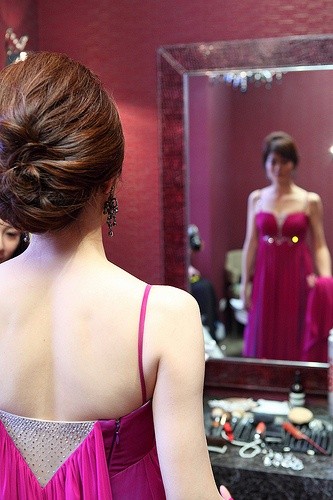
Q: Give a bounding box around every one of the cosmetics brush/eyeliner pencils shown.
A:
[209,406,271,450]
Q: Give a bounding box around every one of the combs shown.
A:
[282,420,330,456]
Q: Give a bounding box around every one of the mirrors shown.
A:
[156,34,332,396]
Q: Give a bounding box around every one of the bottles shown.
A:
[290,369,306,408]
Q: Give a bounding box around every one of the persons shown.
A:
[0,52,235,500]
[0,217,29,264]
[233,129,332,362]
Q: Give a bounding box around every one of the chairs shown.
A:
[222,249,252,333]
